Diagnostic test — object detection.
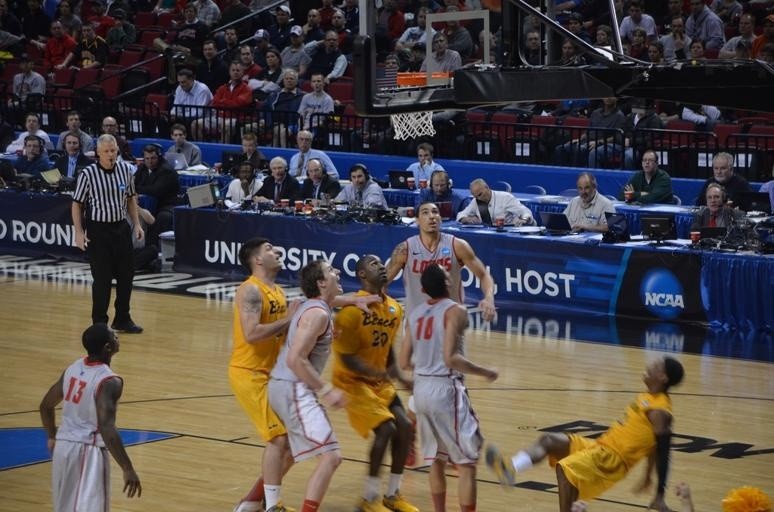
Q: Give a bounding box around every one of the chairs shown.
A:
[0,0,774,164]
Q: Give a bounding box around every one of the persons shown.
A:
[7,53,46,113]
[134,142,189,245]
[396,260,496,511]
[225,161,264,209]
[222,133,266,174]
[289,158,341,206]
[51,132,95,180]
[688,182,738,232]
[233,258,382,512]
[415,170,461,218]
[696,152,753,211]
[7,113,55,154]
[289,130,340,180]
[455,179,533,226]
[484,353,683,511]
[335,163,388,208]
[13,136,49,188]
[56,111,95,153]
[570,482,696,511]
[102,116,135,161]
[379,201,496,470]
[70,134,145,334]
[362,1,774,168]
[331,254,414,512]
[562,171,617,232]
[168,124,202,166]
[2,2,361,152]
[225,237,288,511]
[253,156,299,204]
[37,322,142,511]
[406,143,445,188]
[617,149,674,203]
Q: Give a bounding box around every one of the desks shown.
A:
[0,164,774,338]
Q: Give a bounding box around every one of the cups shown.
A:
[691,232,701,244]
[15,147,23,158]
[406,176,415,190]
[214,163,222,174]
[135,158,143,165]
[419,179,426,191]
[435,202,452,220]
[279,198,312,216]
[405,206,414,218]
[495,216,507,228]
[624,190,635,203]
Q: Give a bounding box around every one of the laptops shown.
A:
[0,158,14,177]
[732,191,772,212]
[540,211,580,233]
[222,151,240,169]
[604,211,643,241]
[387,169,416,189]
[164,152,191,170]
[433,201,455,219]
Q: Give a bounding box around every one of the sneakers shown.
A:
[357,493,393,512]
[485,445,516,491]
[95,315,145,335]
[234,497,294,511]
[384,491,421,512]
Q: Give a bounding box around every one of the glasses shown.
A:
[308,167,322,175]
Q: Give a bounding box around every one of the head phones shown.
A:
[359,164,372,180]
[152,142,164,160]
[722,188,730,202]
[23,136,45,152]
[447,174,454,190]
[62,133,82,149]
[285,163,289,172]
[305,157,327,177]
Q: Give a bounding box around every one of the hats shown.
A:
[18,53,33,62]
[254,4,302,39]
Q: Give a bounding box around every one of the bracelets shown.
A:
[316,381,334,399]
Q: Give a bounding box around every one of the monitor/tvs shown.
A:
[639,212,676,247]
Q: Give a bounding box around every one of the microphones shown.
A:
[718,203,724,209]
[73,148,80,152]
[360,181,365,184]
